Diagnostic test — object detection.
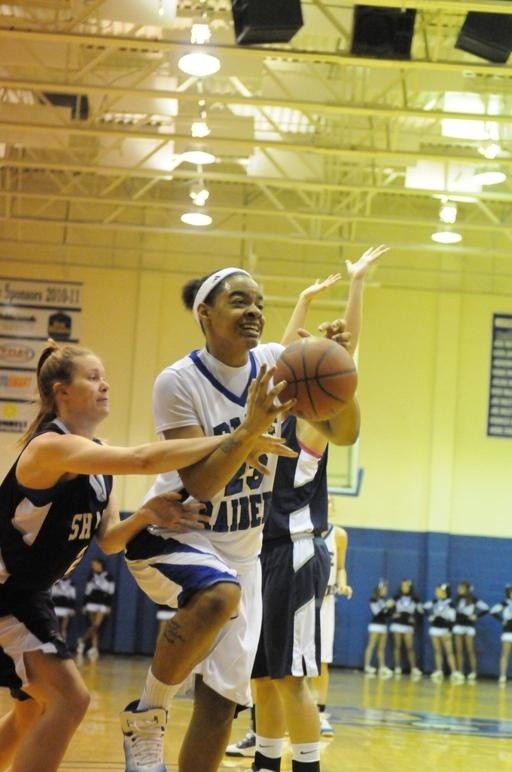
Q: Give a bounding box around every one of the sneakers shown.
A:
[431,669,508,682]
[118,697,170,772]
[318,709,334,737]
[224,729,260,757]
[363,664,422,675]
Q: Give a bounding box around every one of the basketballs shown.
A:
[273,338,357,421]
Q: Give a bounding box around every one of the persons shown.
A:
[363,579,512,684]
[222,524,353,759]
[76,557,116,659]
[120,265,353,772]
[50,570,77,642]
[0,338,298,772]
